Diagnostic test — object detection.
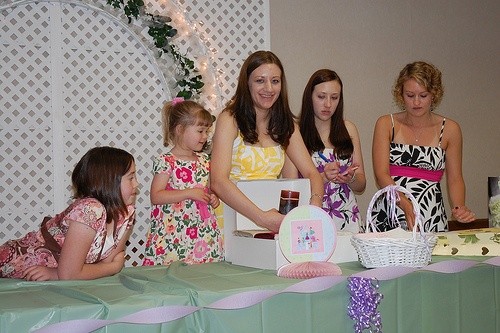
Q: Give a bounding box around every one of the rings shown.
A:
[454,206,460,210]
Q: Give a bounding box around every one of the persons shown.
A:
[0,147,139,281]
[372,61,477,232]
[143,97,225,266]
[282,68,366,233]
[210,51,325,233]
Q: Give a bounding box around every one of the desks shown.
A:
[0,248,499,333]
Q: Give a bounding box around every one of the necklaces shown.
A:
[413,136,424,141]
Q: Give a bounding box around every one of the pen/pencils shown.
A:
[319,152,330,163]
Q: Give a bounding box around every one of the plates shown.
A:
[279,205,337,263]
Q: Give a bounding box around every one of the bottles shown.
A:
[278,189,300,214]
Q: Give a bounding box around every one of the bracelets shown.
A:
[347,174,355,185]
[311,192,323,200]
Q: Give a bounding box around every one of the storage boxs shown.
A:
[222,179,361,272]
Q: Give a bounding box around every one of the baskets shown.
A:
[351,185,438,270]
